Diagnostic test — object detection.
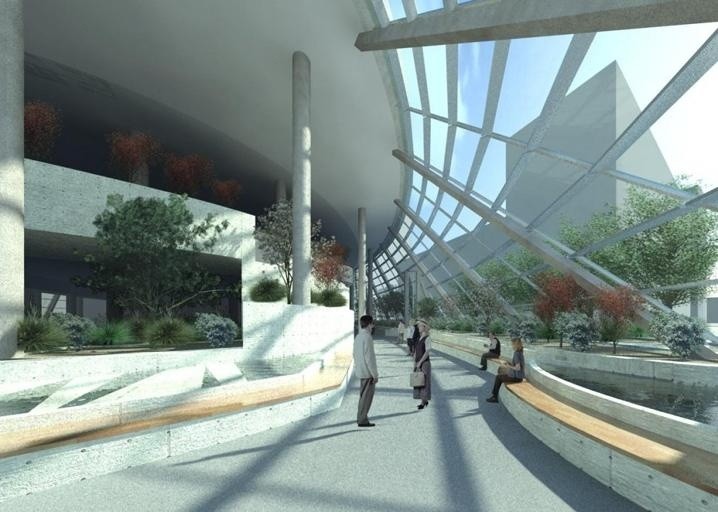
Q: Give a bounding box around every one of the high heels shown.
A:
[418,401,428,409]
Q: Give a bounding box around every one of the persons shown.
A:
[478,331,500,370]
[406,319,420,356]
[409,319,432,409]
[487,338,525,403]
[396,320,406,344]
[353,315,379,427]
[371,322,376,338]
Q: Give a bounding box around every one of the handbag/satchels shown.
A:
[410,368,425,387]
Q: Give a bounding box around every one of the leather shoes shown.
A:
[358,424,375,426]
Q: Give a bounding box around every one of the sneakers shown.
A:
[487,397,498,402]
[480,367,486,369]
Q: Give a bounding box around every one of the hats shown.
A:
[416,319,427,327]
